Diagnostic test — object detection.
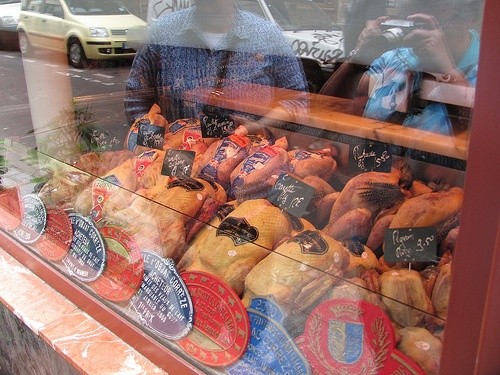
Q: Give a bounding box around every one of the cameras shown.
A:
[376,19,415,51]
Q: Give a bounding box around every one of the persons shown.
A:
[18,29,81,175]
[318,1,482,160]
[344,1,386,61]
[123,0,312,182]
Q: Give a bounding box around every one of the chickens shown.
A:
[38,104,465,375]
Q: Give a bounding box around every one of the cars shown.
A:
[16,0,149,68]
[0,0,21,49]
[147,0,347,93]
